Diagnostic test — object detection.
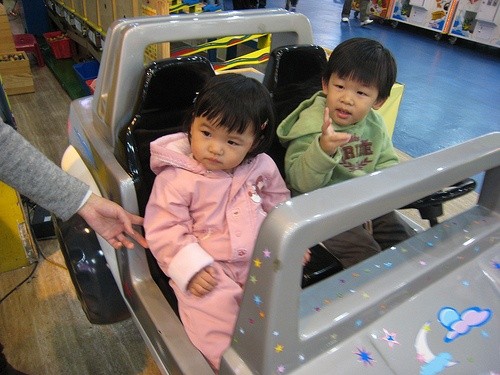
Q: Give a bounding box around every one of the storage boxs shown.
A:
[72,60,99,95]
[43,31,72,59]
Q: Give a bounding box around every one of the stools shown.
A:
[14,34,45,67]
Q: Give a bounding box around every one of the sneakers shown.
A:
[342,17,349,22]
[360,19,373,26]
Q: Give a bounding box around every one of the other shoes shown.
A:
[285,5,289,10]
[291,6,296,12]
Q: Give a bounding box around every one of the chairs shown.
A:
[117,54,309,300]
[261,44,344,284]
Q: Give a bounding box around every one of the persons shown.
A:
[143,72,312,370]
[258,0,298,12]
[0,117,151,375]
[276,37,414,268]
[341,0,374,26]
